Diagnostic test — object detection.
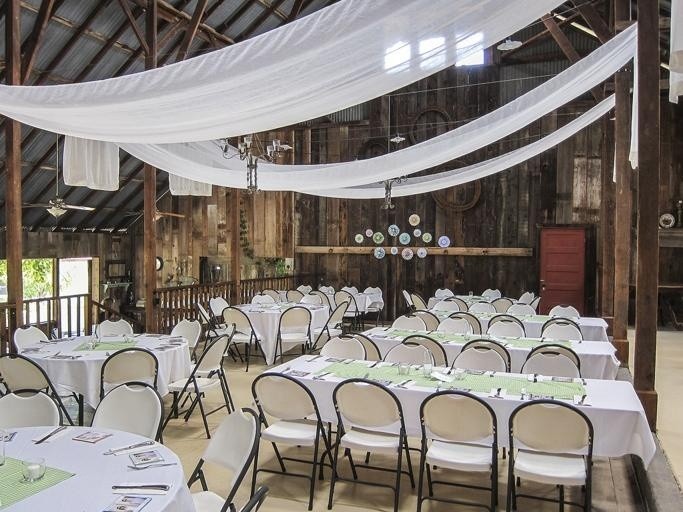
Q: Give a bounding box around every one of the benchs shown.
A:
[615,277,683,328]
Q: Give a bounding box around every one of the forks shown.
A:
[125,462,178,471]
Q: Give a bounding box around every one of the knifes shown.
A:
[277,353,503,399]
[104,439,154,455]
[112,483,170,492]
[34,424,68,444]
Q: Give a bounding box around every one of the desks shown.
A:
[630,226,682,282]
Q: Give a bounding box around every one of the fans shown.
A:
[19,130,185,227]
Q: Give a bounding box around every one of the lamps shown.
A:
[217,134,292,194]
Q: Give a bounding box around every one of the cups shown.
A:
[0,427,6,467]
[23,458,45,481]
[468,291,472,302]
[396,348,466,379]
[86,323,99,351]
